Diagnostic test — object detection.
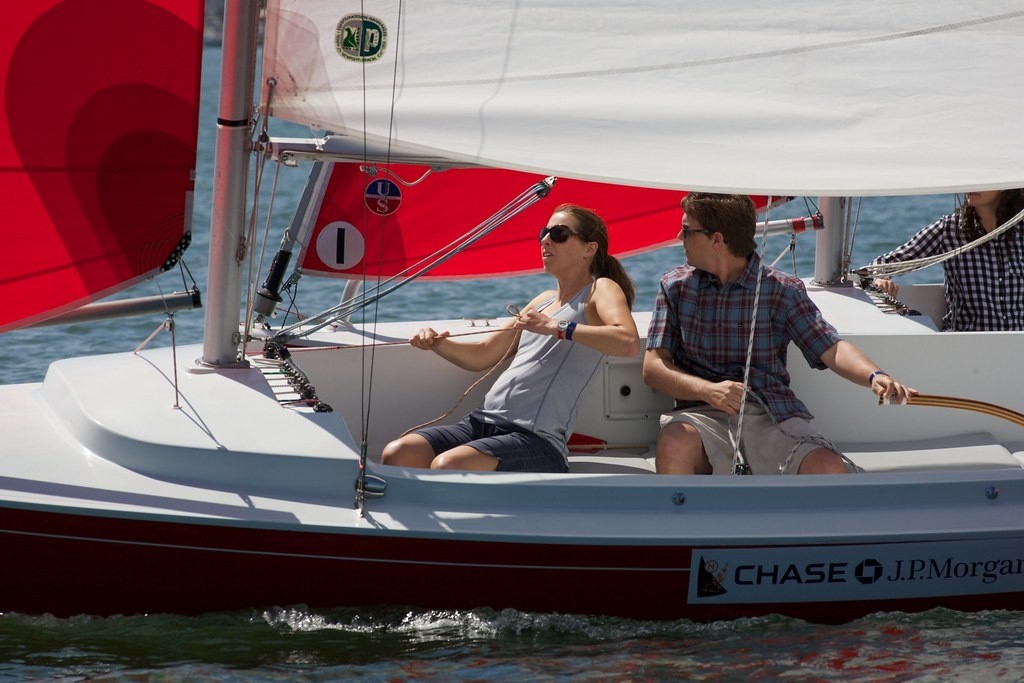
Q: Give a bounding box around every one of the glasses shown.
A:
[540,225,595,245]
[681,225,727,244]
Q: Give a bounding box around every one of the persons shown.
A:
[381,204,641,473]
[643,192,919,474]
[870,188,1024,332]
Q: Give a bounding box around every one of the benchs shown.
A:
[567,431,1022,475]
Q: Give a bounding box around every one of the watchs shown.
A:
[557,320,568,339]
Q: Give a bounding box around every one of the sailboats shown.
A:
[0,1,1024,618]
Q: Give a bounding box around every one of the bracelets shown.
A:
[869,371,890,385]
[566,322,577,341]
